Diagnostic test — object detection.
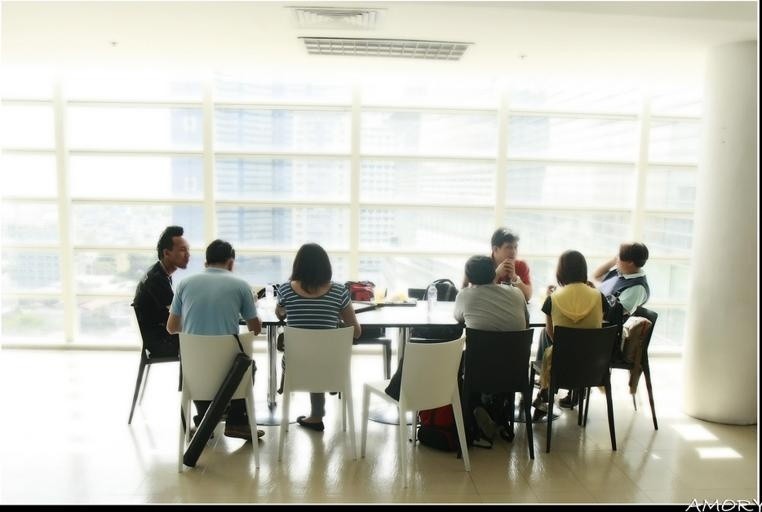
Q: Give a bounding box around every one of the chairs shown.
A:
[278,326,357,463]
[408,288,459,302]
[352,288,392,380]
[361,328,472,489]
[128,303,182,425]
[178,331,260,474]
[456,328,535,460]
[570,307,658,430]
[529,325,618,454]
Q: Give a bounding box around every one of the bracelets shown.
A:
[513,274,521,286]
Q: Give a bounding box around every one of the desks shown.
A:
[239,296,610,427]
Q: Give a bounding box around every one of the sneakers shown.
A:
[558,385,591,408]
[533,388,549,408]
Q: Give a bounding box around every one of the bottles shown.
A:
[426,278,438,316]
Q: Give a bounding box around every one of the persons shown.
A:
[135,227,188,361]
[491,230,531,301]
[275,245,362,431]
[534,254,603,424]
[167,243,265,439]
[596,240,648,323]
[451,257,531,444]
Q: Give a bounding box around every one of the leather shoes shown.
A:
[297,415,325,432]
[224,424,265,439]
[190,427,215,441]
